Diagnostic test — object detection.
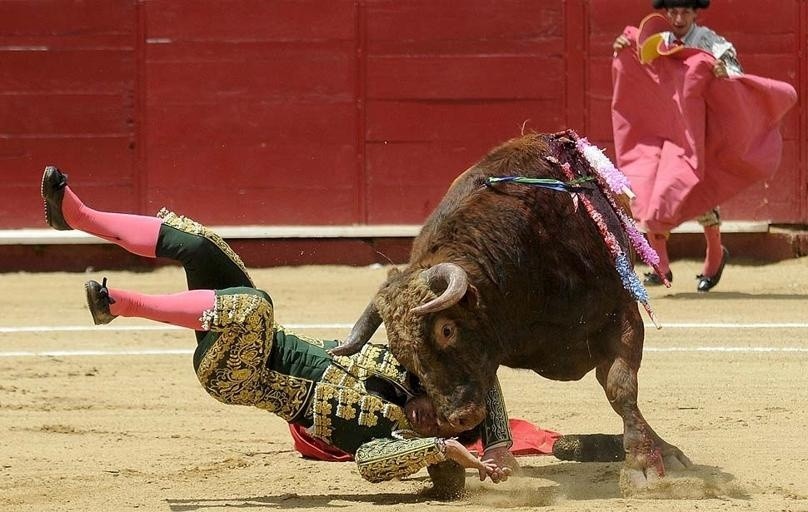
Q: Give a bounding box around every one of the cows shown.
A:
[327,119,691,502]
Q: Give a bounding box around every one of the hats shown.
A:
[650,0,711,9]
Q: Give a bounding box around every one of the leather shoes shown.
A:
[40,165,75,231]
[642,268,673,287]
[85,279,119,327]
[698,244,730,290]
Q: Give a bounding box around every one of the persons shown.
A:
[611,3,745,293]
[39,163,526,484]
